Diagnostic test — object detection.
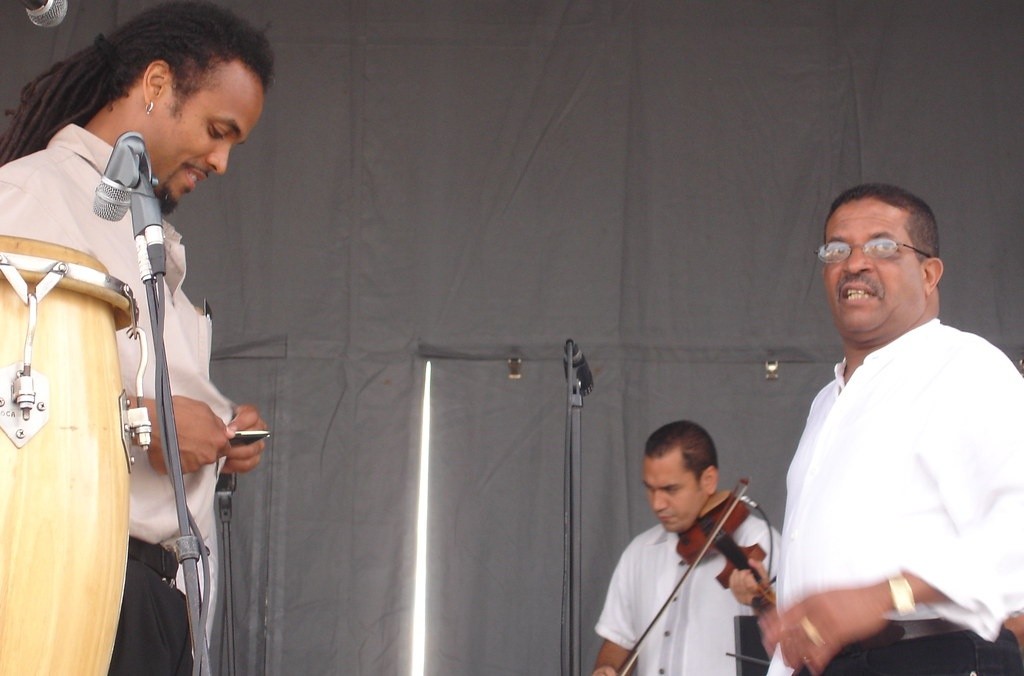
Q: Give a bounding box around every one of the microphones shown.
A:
[563,340,594,396]
[93,132,144,222]
[20,0,68,26]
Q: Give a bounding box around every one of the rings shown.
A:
[801,656,811,665]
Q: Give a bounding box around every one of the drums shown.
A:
[0,233,138,676]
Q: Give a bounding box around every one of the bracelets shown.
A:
[886,573,916,618]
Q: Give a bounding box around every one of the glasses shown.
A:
[812,238,932,264]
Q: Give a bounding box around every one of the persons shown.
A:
[757,182,1024,676]
[1,0,266,676]
[590,419,783,676]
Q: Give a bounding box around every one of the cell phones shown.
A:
[228,430,271,446]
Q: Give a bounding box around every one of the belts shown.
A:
[126,528,179,580]
[840,618,976,658]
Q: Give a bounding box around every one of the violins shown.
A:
[675,488,777,611]
